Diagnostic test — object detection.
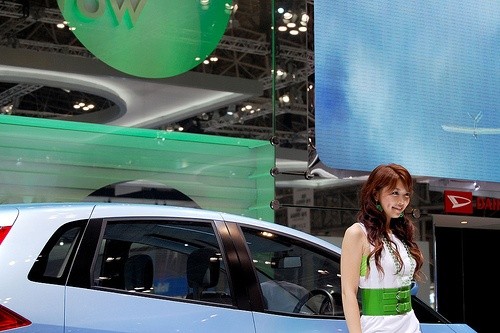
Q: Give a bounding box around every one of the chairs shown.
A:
[183,248,231,304]
[122,252,155,297]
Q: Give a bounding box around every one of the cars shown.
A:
[0,202,477,333]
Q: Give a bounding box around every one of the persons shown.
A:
[341,164,424,333]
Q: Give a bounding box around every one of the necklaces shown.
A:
[384,232,413,284]
